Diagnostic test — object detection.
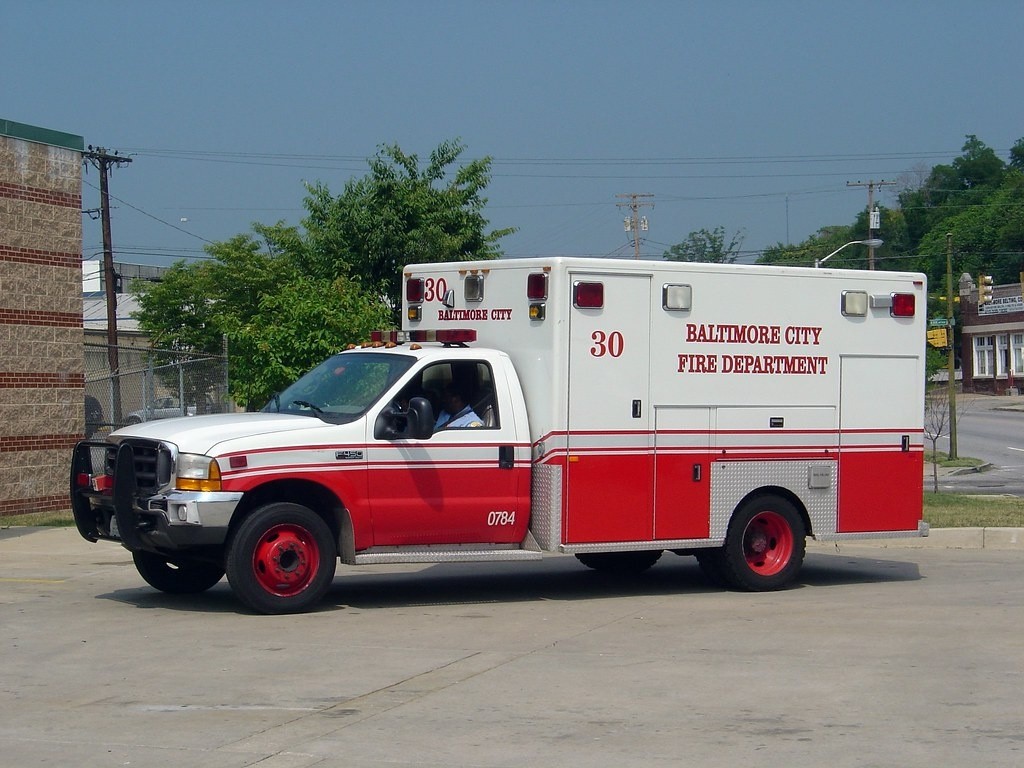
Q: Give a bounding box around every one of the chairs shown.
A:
[471,381,497,427]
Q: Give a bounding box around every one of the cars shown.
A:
[123,391,216,429]
[85,395,106,439]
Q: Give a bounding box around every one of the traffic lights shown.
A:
[978,276,995,305]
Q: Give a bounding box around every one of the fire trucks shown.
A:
[68,255,932,617]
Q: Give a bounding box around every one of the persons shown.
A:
[426,380,486,437]
